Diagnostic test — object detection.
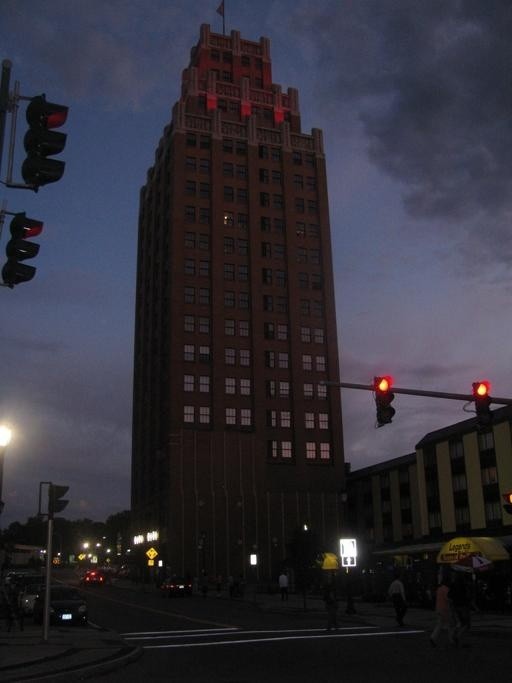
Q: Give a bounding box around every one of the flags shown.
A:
[216,0,224,16]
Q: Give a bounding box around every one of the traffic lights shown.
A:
[21,93,68,192]
[372,374,395,426]
[47,484,70,515]
[501,491,512,515]
[2,213,44,289]
[471,381,494,436]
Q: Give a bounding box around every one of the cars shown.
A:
[157,573,194,599]
[5,569,89,627]
[78,568,105,587]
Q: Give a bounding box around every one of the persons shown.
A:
[324,577,339,632]
[344,578,356,615]
[453,570,476,648]
[427,579,454,646]
[277,570,289,601]
[6,571,25,632]
[389,576,409,630]
[199,574,248,599]
